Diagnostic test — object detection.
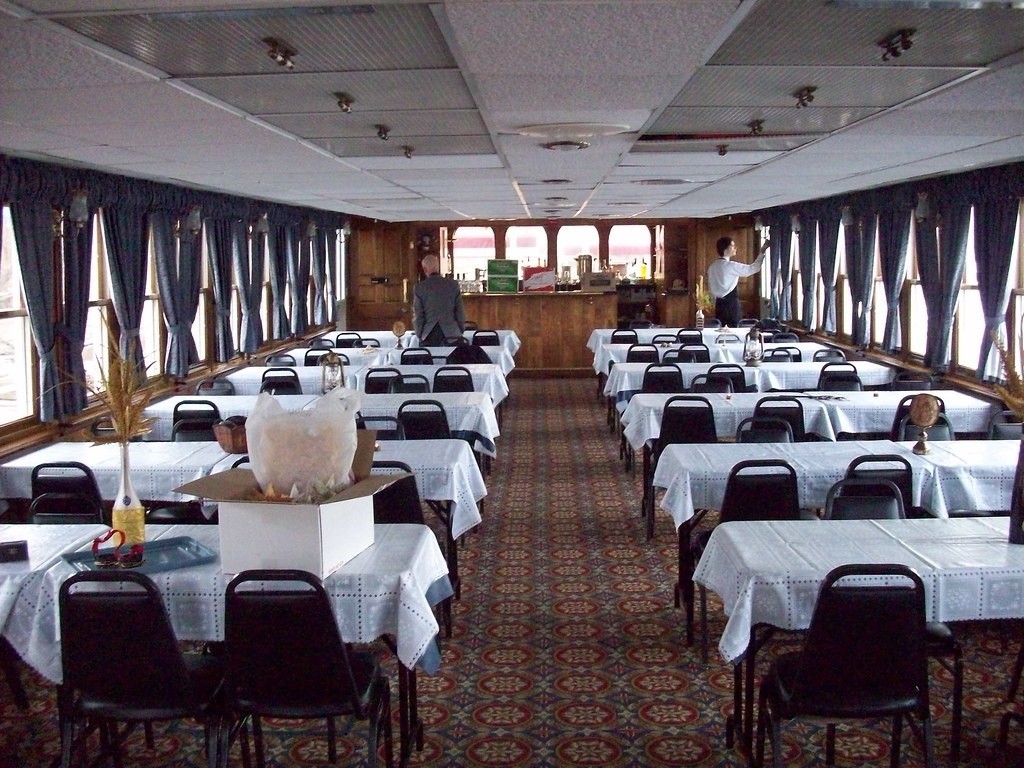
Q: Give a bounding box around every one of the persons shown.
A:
[706,237,773,328]
[412,254,466,348]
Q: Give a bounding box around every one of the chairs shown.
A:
[587,315,1024,768]
[1,320,525,768]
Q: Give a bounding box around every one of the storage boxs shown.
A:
[488,259,518,277]
[487,277,519,294]
[523,267,555,291]
[171,429,420,582]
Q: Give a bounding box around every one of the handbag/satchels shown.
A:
[245,384,364,497]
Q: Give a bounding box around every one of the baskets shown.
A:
[212,414,249,454]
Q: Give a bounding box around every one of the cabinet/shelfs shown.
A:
[344,222,415,331]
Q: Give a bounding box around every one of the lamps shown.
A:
[743,328,765,366]
[302,220,317,242]
[838,201,865,229]
[51,187,90,241]
[790,210,812,236]
[172,200,202,237]
[245,214,270,243]
[753,213,773,234]
[321,350,345,394]
[914,192,943,232]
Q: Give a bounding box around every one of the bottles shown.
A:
[615,270,622,285]
[455,273,462,292]
[461,273,465,284]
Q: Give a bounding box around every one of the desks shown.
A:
[354,364,510,433]
[602,362,761,473]
[1,441,231,526]
[717,342,830,362]
[0,526,456,768]
[225,366,364,397]
[0,524,112,710]
[804,390,992,440]
[320,331,414,348]
[303,392,502,513]
[141,395,318,440]
[592,343,728,433]
[895,441,1021,519]
[618,393,837,543]
[409,329,522,358]
[280,348,393,367]
[387,347,516,403]
[201,440,487,640]
[648,439,936,647]
[688,515,1024,768]
[587,327,705,398]
[756,360,896,393]
[699,326,774,344]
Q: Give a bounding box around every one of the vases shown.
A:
[1007,422,1024,544]
